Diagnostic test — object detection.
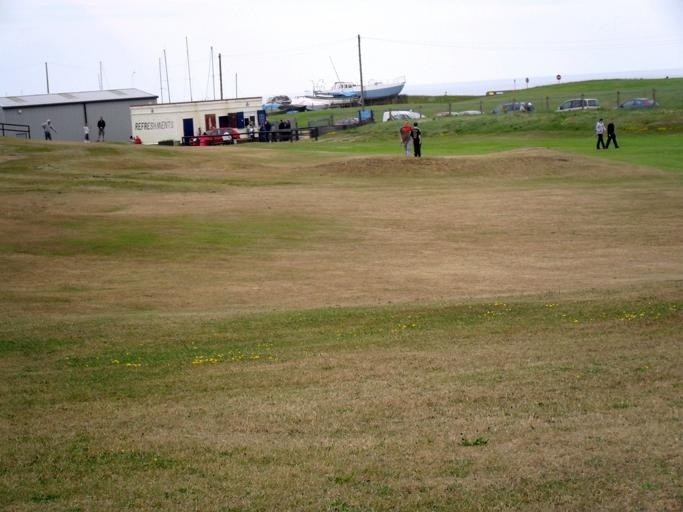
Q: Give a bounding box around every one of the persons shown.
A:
[399,120,422,159]
[198,127,201,135]
[247,119,298,142]
[41,119,57,140]
[520,100,533,112]
[128,136,142,144]
[97,116,106,142]
[82,125,91,143]
[595,118,620,149]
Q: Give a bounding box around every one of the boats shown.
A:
[263,80,406,114]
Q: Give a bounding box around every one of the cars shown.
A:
[189,128,241,146]
[383,97,536,127]
[553,98,600,113]
[616,98,659,111]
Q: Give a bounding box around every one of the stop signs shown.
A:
[556,75,561,80]
